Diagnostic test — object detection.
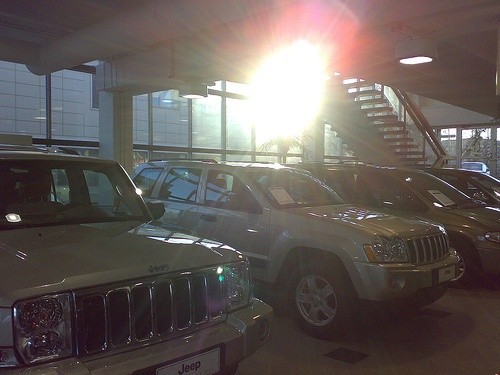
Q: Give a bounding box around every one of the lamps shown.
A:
[395,38,438,65]
[179,83,208,98]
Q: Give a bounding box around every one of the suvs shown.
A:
[112,157,460,341]
[0,139,277,375]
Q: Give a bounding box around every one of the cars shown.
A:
[274,160,500,293]
